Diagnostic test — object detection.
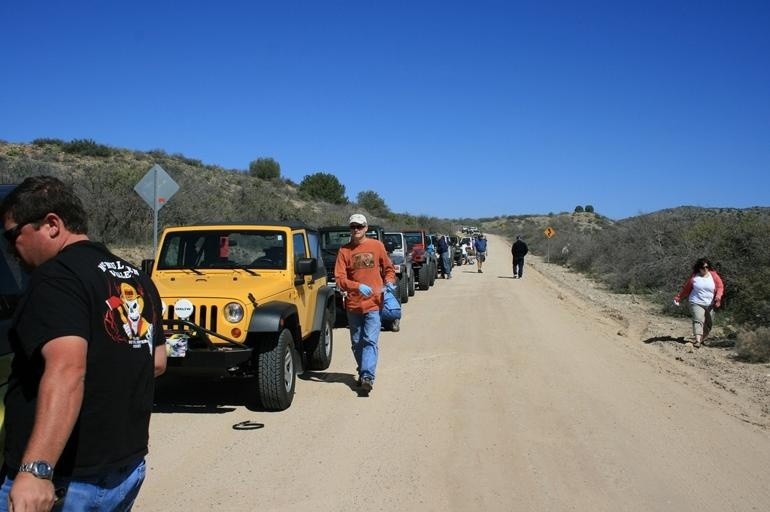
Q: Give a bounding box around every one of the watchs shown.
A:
[17,460,54,480]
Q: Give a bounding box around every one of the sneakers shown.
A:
[392,319,400,332]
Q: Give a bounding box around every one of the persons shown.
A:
[0,174,167,512]
[673,258,724,348]
[380,291,401,332]
[511,235,528,279]
[434,231,452,279]
[474,234,488,273]
[334,214,397,393]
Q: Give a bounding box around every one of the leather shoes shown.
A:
[361,376,373,393]
[356,375,361,387]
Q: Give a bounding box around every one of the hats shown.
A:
[349,213,367,226]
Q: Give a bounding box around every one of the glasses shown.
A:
[700,266,709,270]
[350,225,364,230]
[5,219,27,241]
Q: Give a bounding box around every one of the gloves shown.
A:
[360,284,372,297]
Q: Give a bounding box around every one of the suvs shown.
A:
[317,223,480,326]
[142,223,336,412]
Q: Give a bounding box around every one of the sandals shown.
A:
[693,341,701,348]
